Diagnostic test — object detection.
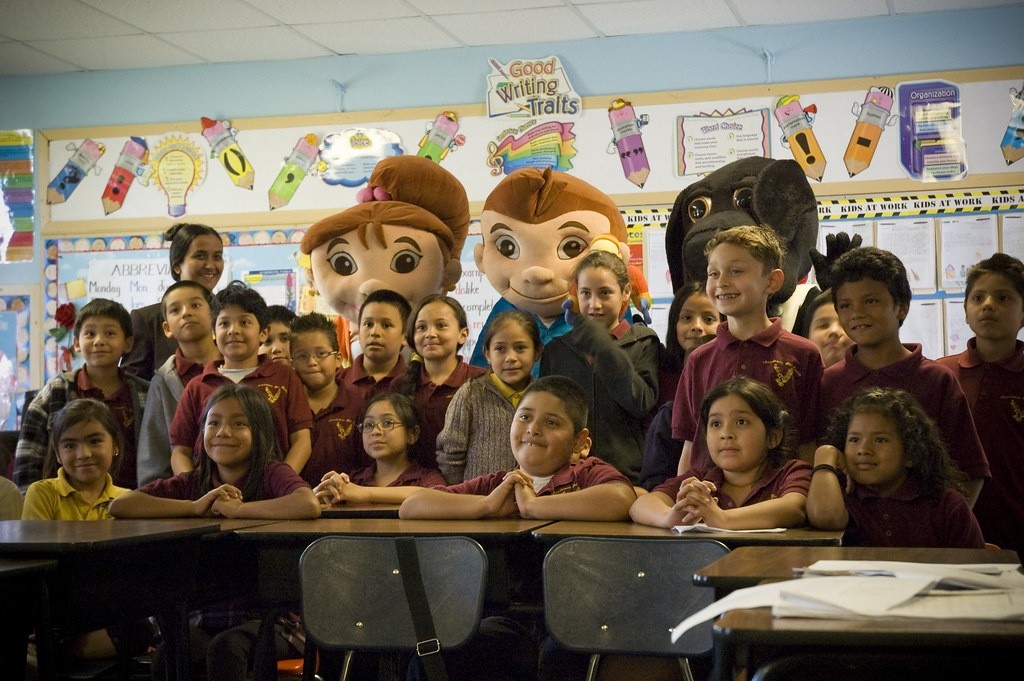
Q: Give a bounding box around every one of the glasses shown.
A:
[355,420,405,433]
[293,349,339,363]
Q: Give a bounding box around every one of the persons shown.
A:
[0,224,991,681]
[935,253,1024,550]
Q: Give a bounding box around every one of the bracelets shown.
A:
[811,464,839,476]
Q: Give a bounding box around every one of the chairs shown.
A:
[298,536,487,681]
[539,539,735,680]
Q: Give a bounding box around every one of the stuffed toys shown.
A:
[301,155,470,369]
[469,168,651,381]
[666,155,862,339]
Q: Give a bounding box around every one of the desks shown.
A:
[0,512,1024,680]
